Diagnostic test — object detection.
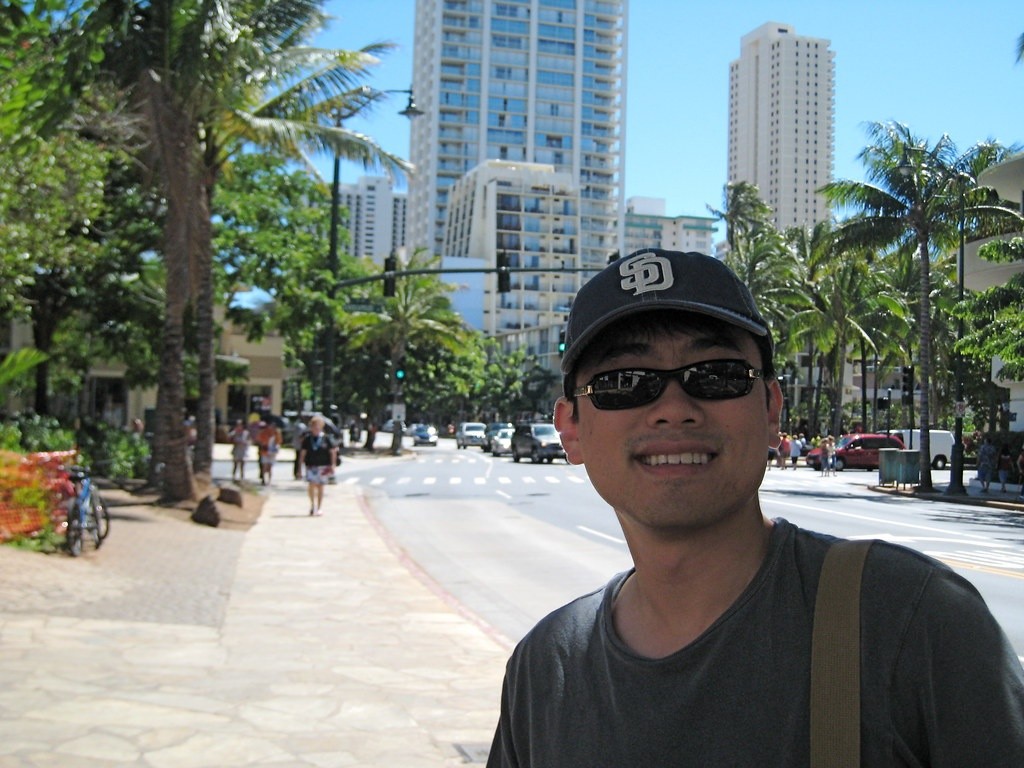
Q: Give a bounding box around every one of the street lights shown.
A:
[898,143,970,494]
[322,88,425,419]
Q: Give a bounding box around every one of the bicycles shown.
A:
[64,459,110,557]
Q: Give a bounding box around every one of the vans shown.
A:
[876,429,955,469]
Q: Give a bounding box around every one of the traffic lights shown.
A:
[901,366,913,392]
[558,329,566,357]
[397,366,406,384]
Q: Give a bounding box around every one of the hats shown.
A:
[562,248,774,399]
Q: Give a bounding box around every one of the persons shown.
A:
[995,441,1015,493]
[767,432,806,470]
[976,438,996,492]
[256,418,282,485]
[1016,445,1024,499]
[291,413,337,516]
[227,417,251,479]
[821,434,837,472]
[487,247,1024,768]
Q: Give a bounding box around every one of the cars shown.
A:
[381,418,406,433]
[806,433,907,471]
[481,422,518,457]
[404,425,424,437]
[413,425,438,446]
[511,423,567,465]
[456,422,488,449]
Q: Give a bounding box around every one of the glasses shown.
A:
[573,357,766,411]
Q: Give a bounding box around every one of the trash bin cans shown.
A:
[896,450,920,489]
[878,448,900,486]
